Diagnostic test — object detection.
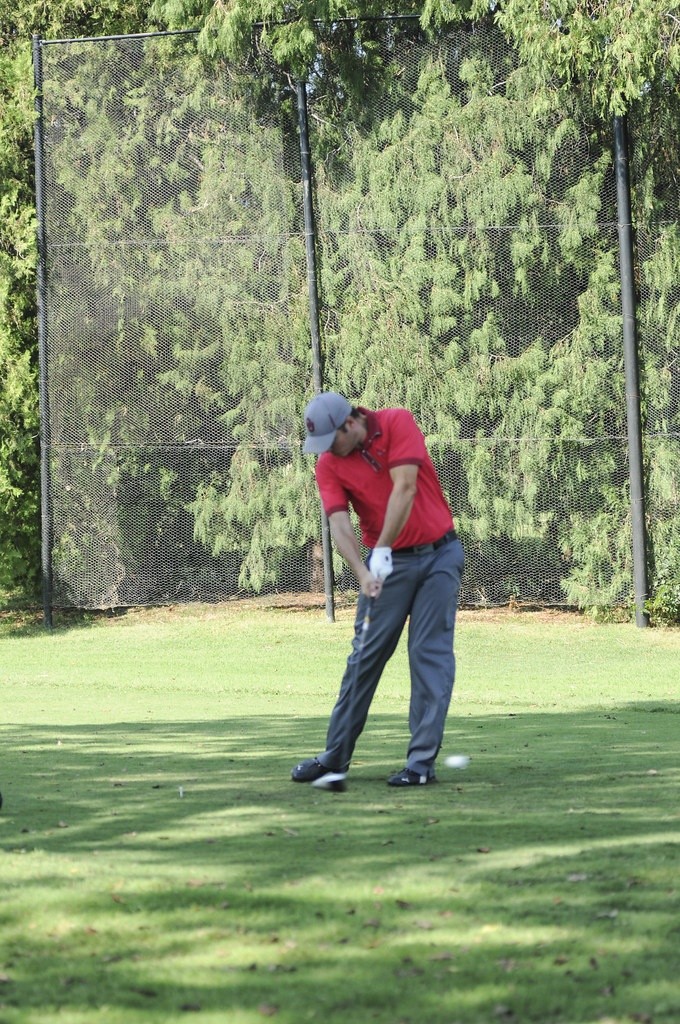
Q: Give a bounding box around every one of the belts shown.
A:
[391,531,458,557]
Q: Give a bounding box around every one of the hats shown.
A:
[302,393,353,453]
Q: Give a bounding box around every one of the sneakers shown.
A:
[291,757,350,783]
[389,769,436,786]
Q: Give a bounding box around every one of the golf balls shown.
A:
[446,754,470,770]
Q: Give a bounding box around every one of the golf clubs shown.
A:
[312,598,371,788]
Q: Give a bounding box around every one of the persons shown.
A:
[287,389,467,787]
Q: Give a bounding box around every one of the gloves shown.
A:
[369,547,393,585]
